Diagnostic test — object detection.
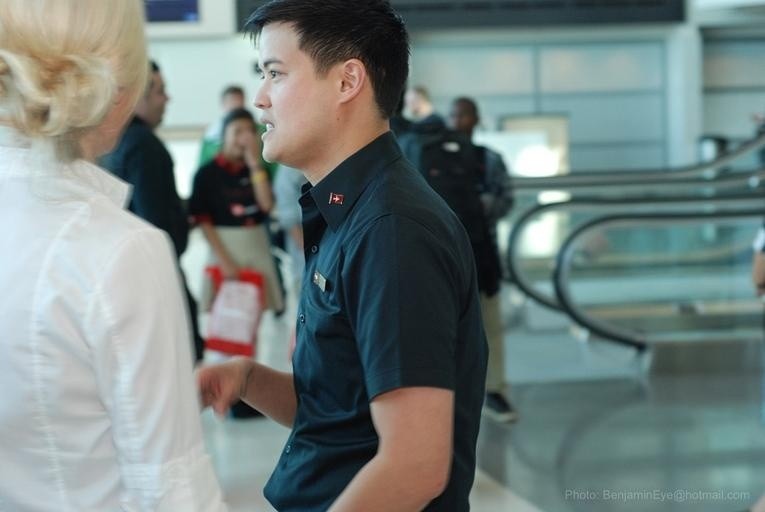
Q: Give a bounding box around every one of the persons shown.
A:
[94,60,515,425]
[198,2,489,512]
[0,1,227,512]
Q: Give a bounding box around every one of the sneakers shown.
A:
[484,393,515,423]
